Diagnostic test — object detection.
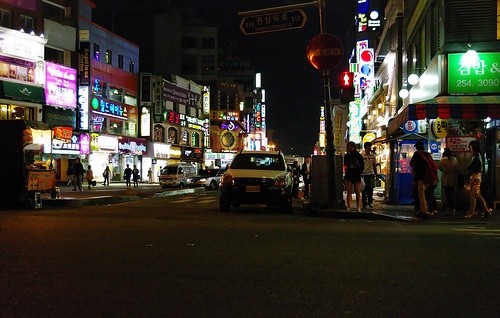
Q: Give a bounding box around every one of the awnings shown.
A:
[0,80,44,102]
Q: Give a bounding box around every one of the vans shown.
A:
[159,163,196,189]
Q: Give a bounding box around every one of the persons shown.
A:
[158,166,163,182]
[124,164,139,188]
[102,166,111,187]
[86,165,94,189]
[344,141,365,213]
[362,142,379,209]
[410,141,438,216]
[292,154,311,201]
[82,168,86,184]
[148,167,153,184]
[198,165,208,175]
[66,157,83,191]
[437,141,491,218]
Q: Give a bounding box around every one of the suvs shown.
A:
[219,147,294,214]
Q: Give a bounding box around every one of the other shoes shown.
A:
[134,186,135,188]
[415,211,430,218]
[357,208,361,212]
[369,203,374,207]
[464,211,478,219]
[481,209,493,218]
[362,206,366,209]
[72,189,77,191]
[79,187,81,189]
[434,210,438,213]
[346,208,350,212]
[430,211,434,215]
[449,209,456,216]
[137,185,138,187]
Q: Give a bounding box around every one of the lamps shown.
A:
[462,43,479,67]
[399,84,411,98]
[408,66,426,85]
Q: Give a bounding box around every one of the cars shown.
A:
[196,167,226,191]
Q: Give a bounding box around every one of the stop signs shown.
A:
[306,33,344,71]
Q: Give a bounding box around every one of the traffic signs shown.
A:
[238,8,308,35]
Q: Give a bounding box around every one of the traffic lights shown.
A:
[340,86,355,103]
[339,71,354,89]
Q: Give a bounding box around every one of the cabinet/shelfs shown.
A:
[396,171,415,204]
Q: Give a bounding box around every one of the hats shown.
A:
[414,141,424,146]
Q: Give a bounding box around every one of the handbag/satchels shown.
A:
[92,181,96,186]
[124,176,128,180]
[136,175,141,179]
[432,172,439,185]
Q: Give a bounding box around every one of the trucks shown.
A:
[286,156,306,192]
